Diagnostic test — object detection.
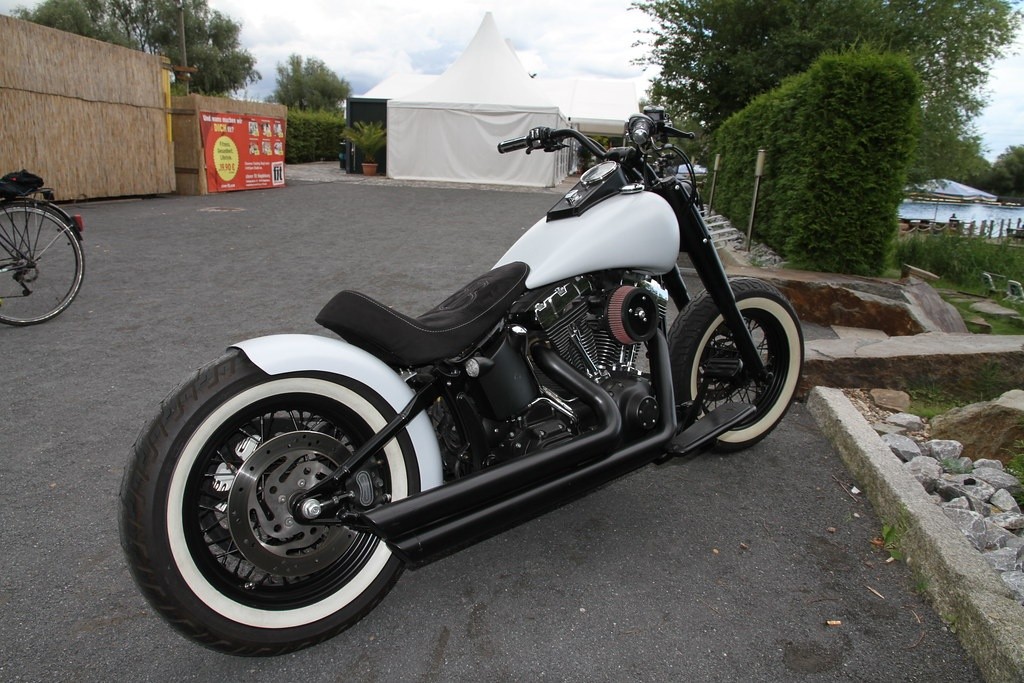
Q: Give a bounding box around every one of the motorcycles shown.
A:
[114,103,806,660]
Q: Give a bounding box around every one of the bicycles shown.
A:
[0,187,86,325]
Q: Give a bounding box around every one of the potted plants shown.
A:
[339,120,386,176]
